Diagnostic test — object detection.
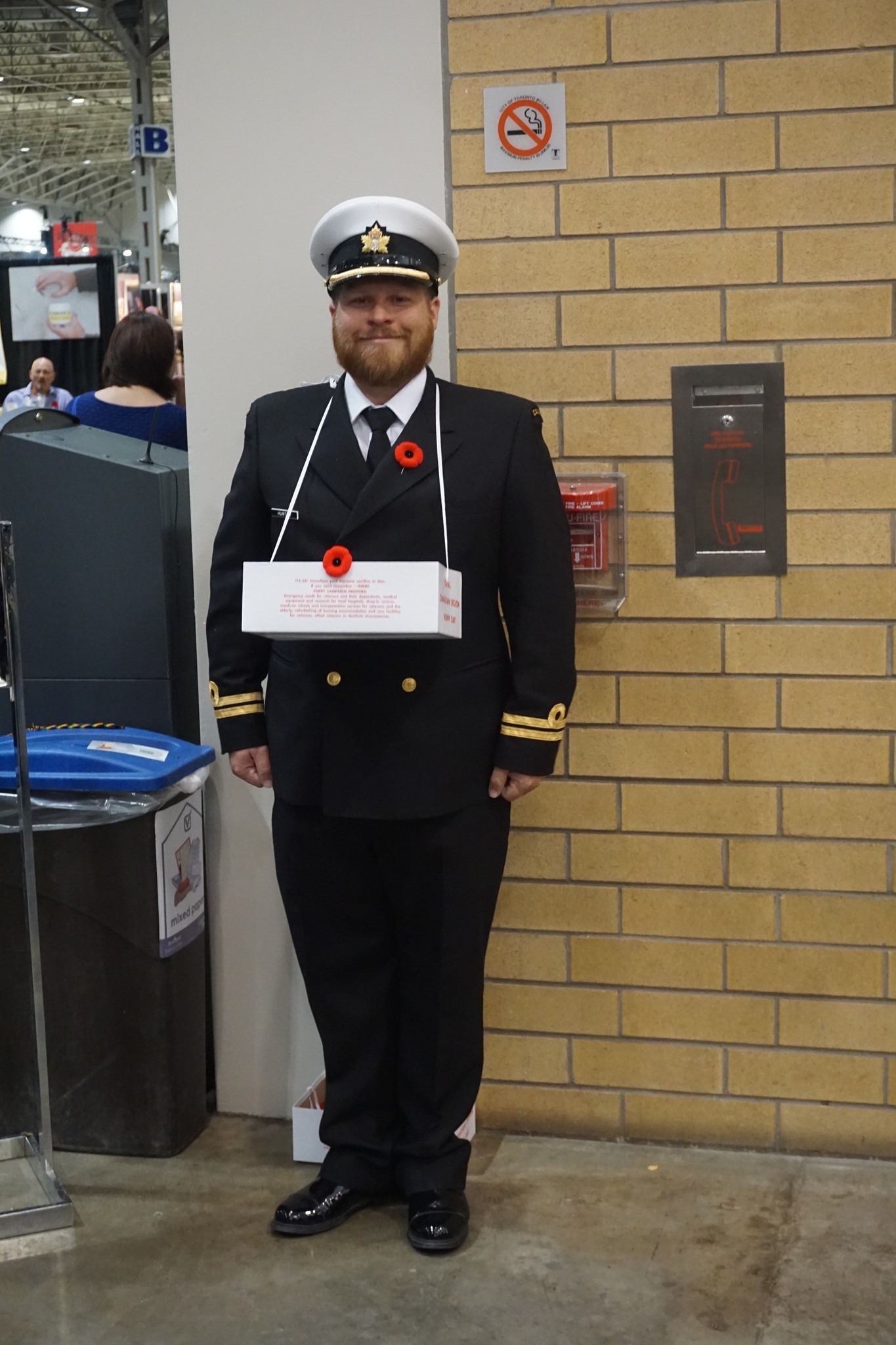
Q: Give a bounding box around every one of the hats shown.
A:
[307,195,459,299]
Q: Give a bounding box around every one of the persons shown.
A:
[3,311,187,450]
[36,267,99,339]
[207,195,582,1251]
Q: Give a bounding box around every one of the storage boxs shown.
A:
[243,559,462,639]
[294,1067,479,1166]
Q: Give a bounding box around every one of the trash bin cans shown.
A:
[1,726,215,1159]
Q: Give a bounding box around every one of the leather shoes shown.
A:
[270,1173,407,1235]
[407,1188,470,1250]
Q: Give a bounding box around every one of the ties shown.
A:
[360,407,397,475]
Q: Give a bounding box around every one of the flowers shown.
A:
[395,440,424,469]
[322,546,354,577]
